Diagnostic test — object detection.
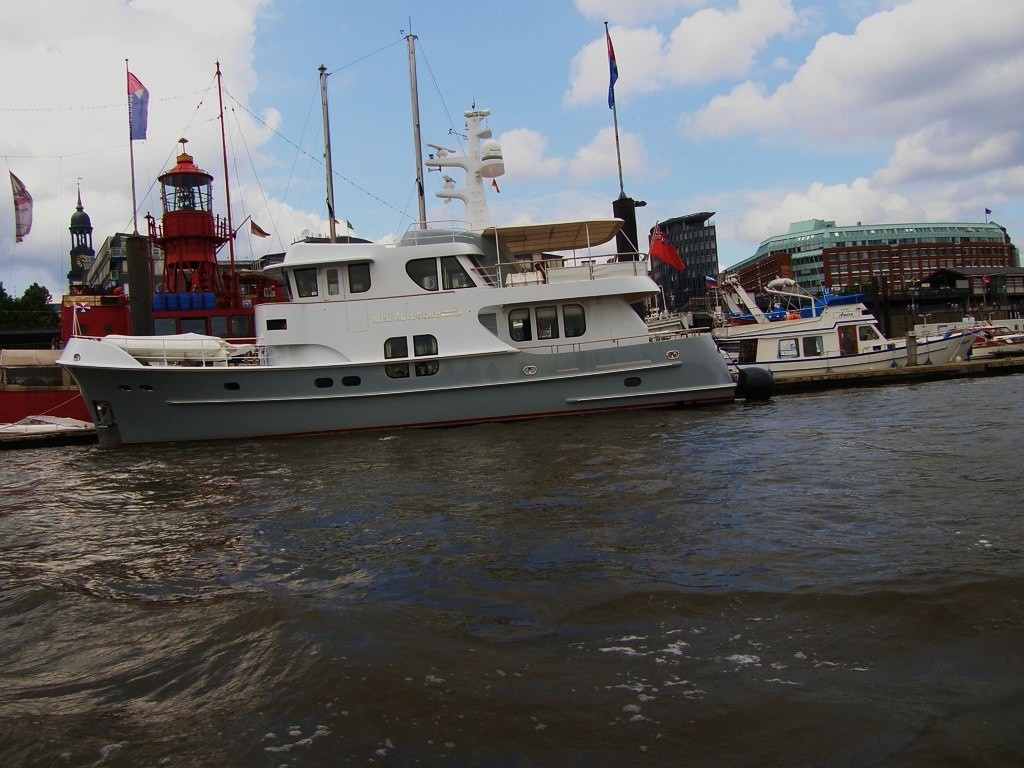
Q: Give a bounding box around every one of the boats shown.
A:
[0,17,1024,451]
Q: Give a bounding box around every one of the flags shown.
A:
[251,220,271,238]
[128,72,149,139]
[985,208,992,214]
[327,202,340,224]
[649,223,686,272]
[605,27,619,109]
[347,221,354,230]
[492,179,500,193]
[706,276,719,289]
[10,171,33,243]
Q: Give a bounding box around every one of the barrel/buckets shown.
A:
[153,293,166,311]
[192,292,203,310]
[203,291,216,310]
[178,292,192,310]
[166,293,178,310]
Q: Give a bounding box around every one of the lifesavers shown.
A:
[157,283,168,292]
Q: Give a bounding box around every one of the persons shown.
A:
[660,308,669,320]
